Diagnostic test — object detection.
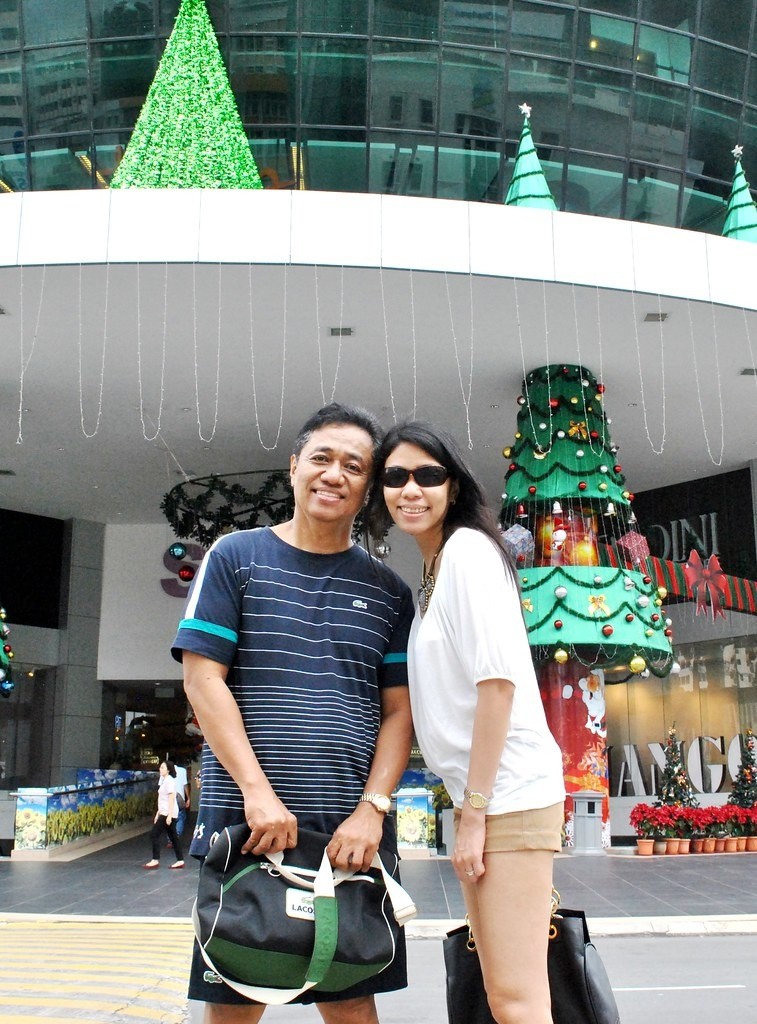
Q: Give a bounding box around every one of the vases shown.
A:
[655,841,667,855]
[748,836,757,852]
[725,838,738,852]
[663,838,679,854]
[635,839,655,856]
[679,838,691,854]
[716,838,726,852]
[703,837,717,853]
[692,839,704,854]
[737,837,747,851]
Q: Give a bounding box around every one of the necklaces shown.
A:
[417,538,443,611]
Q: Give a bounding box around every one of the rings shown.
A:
[466,870,474,876]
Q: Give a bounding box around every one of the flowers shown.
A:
[627,802,757,837]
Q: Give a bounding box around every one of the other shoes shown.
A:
[168,863,184,869]
[166,842,172,848]
[142,863,159,869]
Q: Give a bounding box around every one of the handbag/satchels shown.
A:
[441,887,620,1024]
[188,822,417,1005]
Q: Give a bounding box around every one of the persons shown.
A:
[143,760,185,869]
[171,403,415,1024]
[158,754,190,848]
[367,423,566,1024]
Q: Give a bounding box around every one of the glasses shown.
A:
[379,466,450,488]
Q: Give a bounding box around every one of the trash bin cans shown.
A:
[570,790,605,856]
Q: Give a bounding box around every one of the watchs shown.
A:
[359,793,391,814]
[463,788,492,809]
